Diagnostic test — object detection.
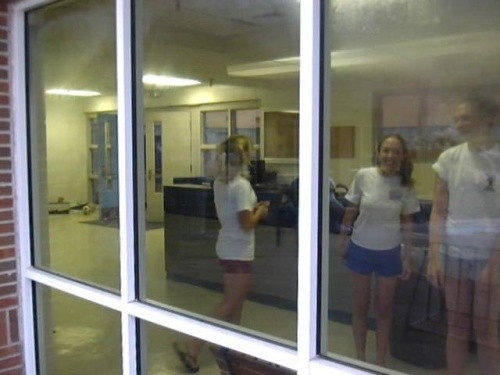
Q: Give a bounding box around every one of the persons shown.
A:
[421,86,500,375]
[336,133,421,367]
[173,134,269,375]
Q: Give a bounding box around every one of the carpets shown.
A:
[78,220,162,232]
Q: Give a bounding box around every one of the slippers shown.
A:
[174,342,198,371]
